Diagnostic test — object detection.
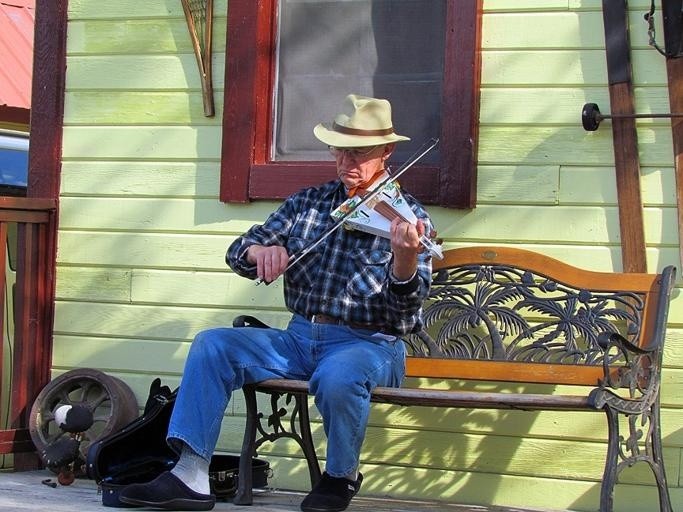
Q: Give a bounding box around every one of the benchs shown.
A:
[231,246,676,512]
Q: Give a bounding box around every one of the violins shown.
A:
[329,169,444,261]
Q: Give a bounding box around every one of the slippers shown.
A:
[299,464,366,512]
[107,465,220,509]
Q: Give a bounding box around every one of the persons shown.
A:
[120,92,435,512]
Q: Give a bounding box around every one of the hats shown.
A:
[312,93,414,148]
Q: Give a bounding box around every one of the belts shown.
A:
[302,313,385,330]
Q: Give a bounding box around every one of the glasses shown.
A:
[327,147,375,159]
